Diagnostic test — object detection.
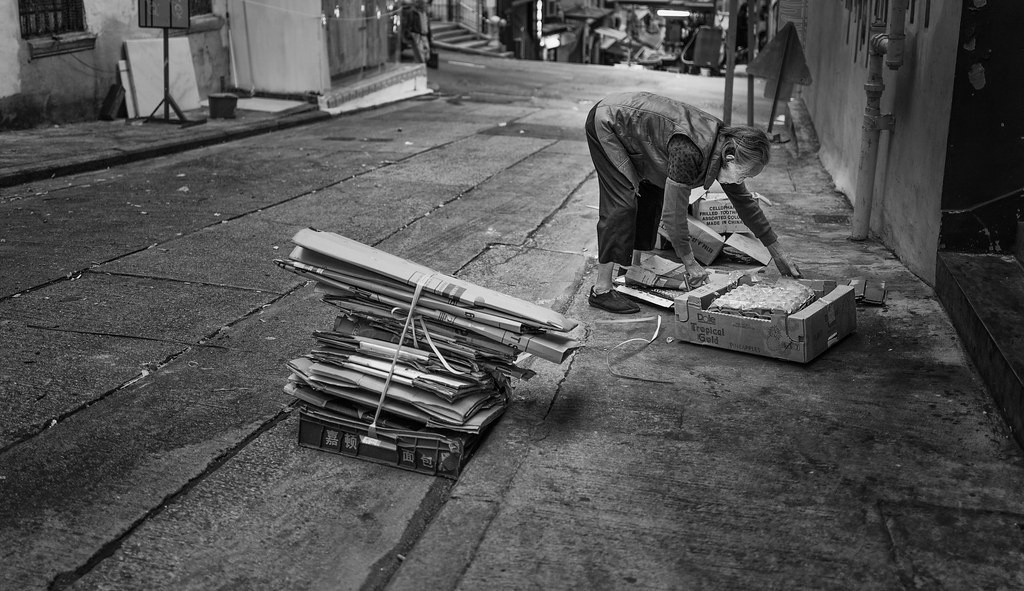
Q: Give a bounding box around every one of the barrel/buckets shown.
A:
[208,93,238,119]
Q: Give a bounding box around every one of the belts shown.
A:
[420,33,429,36]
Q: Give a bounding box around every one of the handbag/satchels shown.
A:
[425,46,439,70]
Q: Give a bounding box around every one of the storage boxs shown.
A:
[658,213,772,267]
[672,274,855,363]
[295,413,463,480]
[687,189,772,235]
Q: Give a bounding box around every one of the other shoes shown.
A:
[589,285,640,314]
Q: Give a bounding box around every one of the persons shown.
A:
[406,0,434,64]
[585,91,803,314]
